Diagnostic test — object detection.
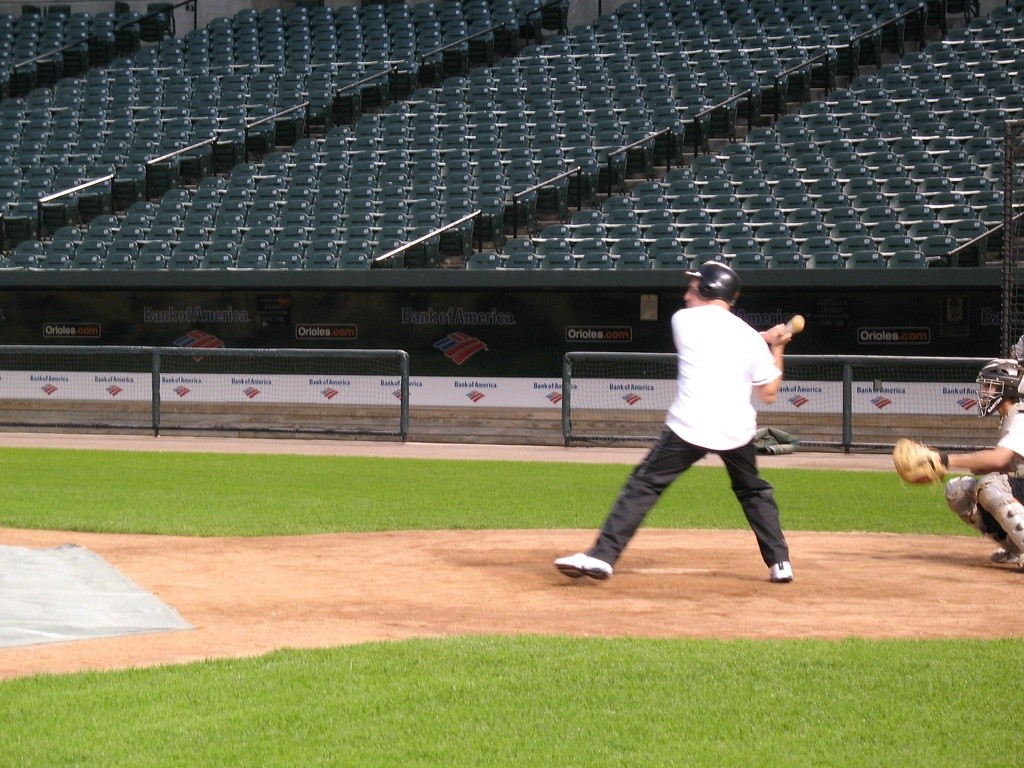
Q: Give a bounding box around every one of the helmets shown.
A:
[975,358,1024,418]
[685,260,738,306]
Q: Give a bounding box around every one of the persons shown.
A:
[892,335,1024,569]
[554,260,792,581]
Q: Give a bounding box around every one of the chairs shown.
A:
[0,0,1024,273]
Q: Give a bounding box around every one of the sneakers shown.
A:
[553,553,612,580]
[769,561,793,581]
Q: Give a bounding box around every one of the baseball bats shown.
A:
[780,314,807,337]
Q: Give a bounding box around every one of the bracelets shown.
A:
[939,452,949,467]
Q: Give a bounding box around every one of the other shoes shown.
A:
[990,546,1021,563]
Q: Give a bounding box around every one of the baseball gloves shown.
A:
[892,437,950,484]
